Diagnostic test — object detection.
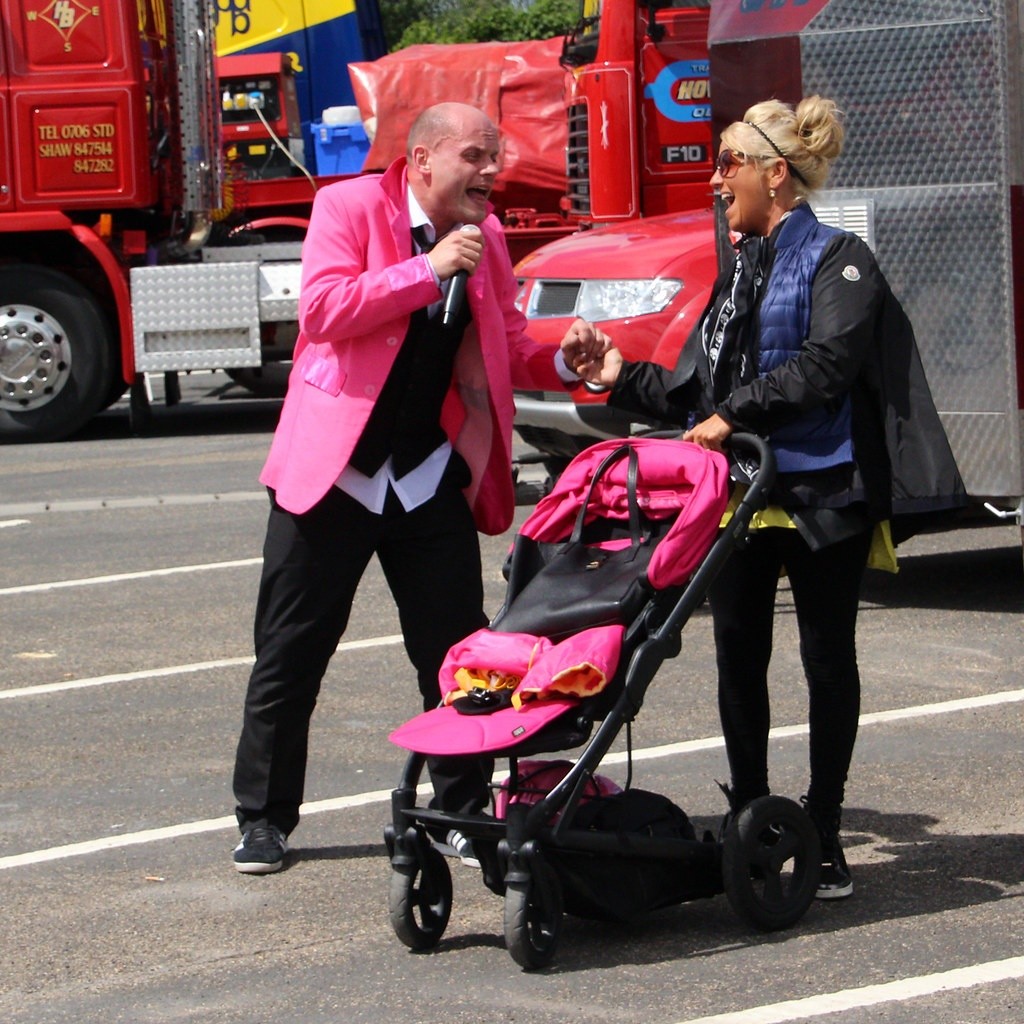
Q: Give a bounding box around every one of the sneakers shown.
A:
[420,821,478,860]
[234,825,285,873]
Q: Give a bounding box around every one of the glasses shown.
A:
[714,150,797,178]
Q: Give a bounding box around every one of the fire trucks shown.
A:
[1,1,1024,576]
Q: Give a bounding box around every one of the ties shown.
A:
[410,225,439,255]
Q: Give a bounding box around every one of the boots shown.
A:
[800,794,853,898]
[713,782,776,848]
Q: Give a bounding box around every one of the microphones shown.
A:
[443,223,481,326]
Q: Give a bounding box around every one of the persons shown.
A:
[572,90,974,902]
[228,98,613,875]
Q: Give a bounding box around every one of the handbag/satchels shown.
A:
[494,760,623,824]
[489,444,680,637]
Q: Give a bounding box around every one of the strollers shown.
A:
[382,430,825,972]
